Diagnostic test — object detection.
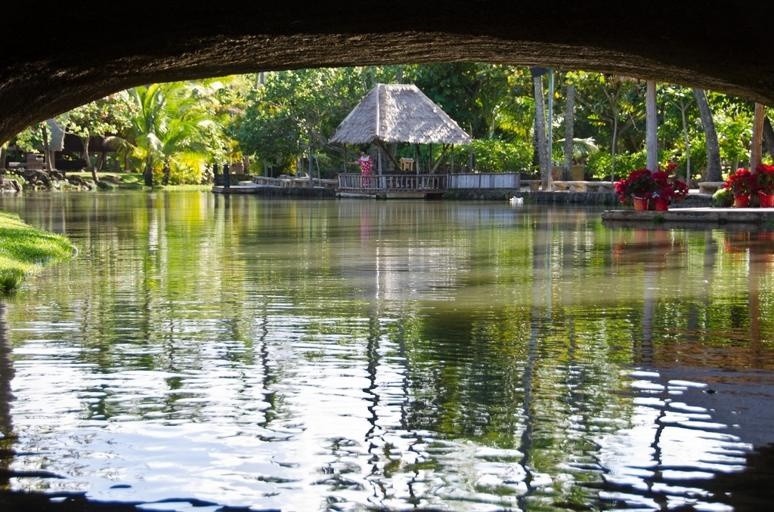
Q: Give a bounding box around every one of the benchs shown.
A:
[249,174,311,188]
[520,179,618,192]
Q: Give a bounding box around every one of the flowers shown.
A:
[720,155,774,201]
[611,156,692,206]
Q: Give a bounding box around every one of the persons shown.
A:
[360,203,372,246]
[346,149,374,189]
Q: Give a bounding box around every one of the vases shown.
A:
[731,190,752,208]
[754,189,774,208]
[652,194,668,212]
[631,191,650,211]
